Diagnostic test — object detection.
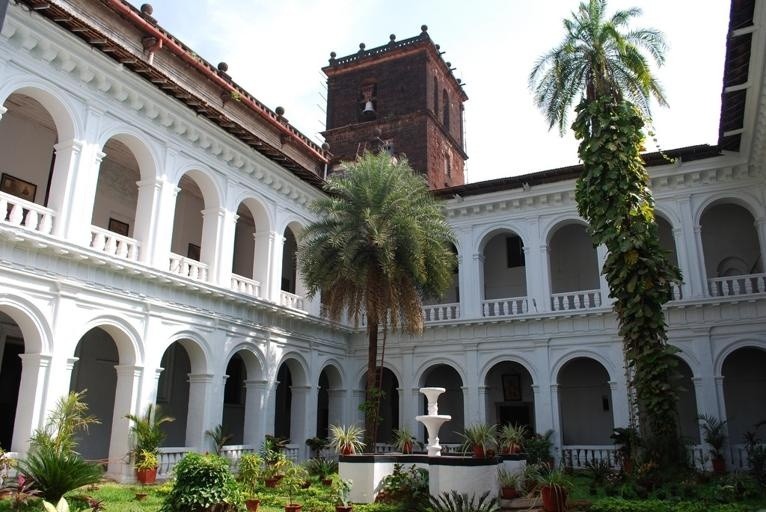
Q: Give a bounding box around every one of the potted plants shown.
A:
[119,401,177,483]
[391,425,425,455]
[237,424,369,512]
[495,457,576,510]
[452,421,528,457]
[695,411,737,475]
[611,425,647,471]
